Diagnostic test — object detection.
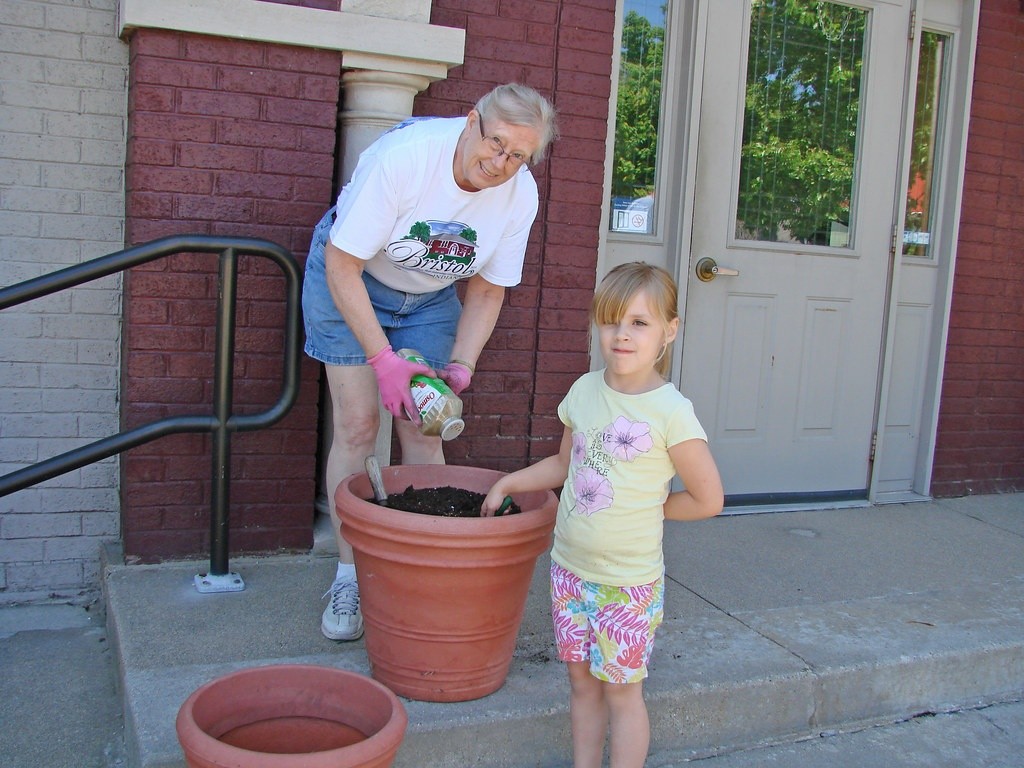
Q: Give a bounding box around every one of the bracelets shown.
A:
[450,360,475,374]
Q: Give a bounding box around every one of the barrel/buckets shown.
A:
[334,464,559,702]
[175,664,406,768]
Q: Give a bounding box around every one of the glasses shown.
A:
[478,113,532,172]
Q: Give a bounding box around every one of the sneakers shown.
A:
[320,574,364,640]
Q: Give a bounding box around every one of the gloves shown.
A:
[366,345,437,427]
[434,362,472,396]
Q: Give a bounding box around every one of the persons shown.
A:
[302,83,554,640]
[478,263,724,768]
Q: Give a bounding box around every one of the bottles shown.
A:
[395,349,465,441]
